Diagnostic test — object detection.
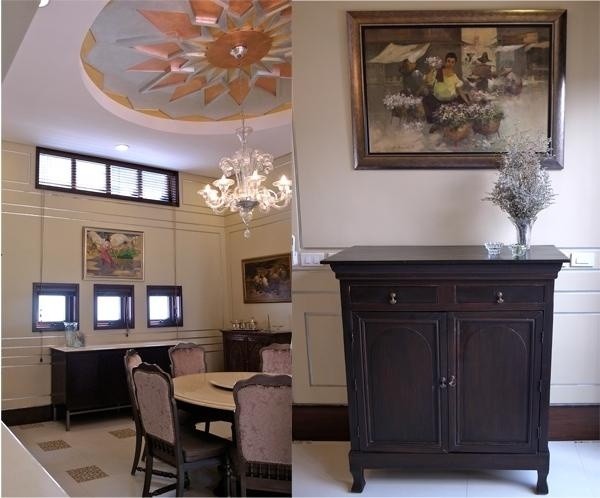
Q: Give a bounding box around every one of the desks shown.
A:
[48,339,178,433]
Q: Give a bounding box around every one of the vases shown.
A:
[510,217,536,253]
[344,9,568,172]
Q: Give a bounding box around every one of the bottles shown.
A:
[73,335,80,348]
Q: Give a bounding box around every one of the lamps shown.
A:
[198,45,293,238]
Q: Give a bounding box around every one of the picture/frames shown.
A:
[240,252,292,305]
[80,226,147,281]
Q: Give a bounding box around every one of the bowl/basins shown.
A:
[485,240,503,254]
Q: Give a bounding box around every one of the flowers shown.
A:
[480,130,558,253]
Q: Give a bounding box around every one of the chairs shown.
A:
[121,341,292,497]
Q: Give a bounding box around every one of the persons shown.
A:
[468,51,499,91]
[99,238,114,266]
[421,52,471,134]
[398,59,426,96]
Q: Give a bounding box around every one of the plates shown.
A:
[208,377,239,389]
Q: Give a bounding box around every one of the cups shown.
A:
[512,244,526,256]
[63,321,78,347]
[231,319,258,330]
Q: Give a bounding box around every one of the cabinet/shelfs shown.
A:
[220,328,292,373]
[319,247,570,494]
